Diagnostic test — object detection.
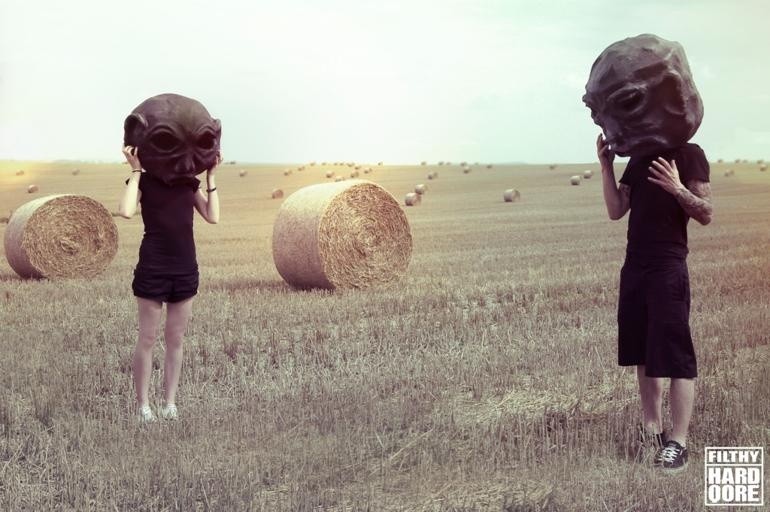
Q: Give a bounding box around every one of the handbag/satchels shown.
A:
[131,168,144,173]
[205,186,218,193]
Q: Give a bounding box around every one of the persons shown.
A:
[106,92,222,424]
[580,30,717,475]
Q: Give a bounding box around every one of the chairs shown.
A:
[164,404,177,420]
[641,432,688,472]
[140,407,156,423]
